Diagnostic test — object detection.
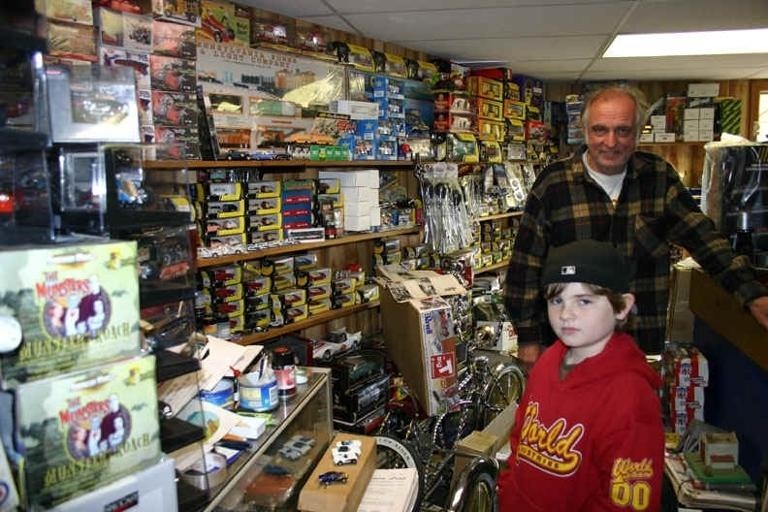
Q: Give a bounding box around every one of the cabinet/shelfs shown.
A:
[157,362,331,511]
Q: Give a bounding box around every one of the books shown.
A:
[665,450,759,512]
[356,467,419,512]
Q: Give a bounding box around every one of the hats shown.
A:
[539,239,630,290]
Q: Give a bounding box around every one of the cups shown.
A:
[198,372,278,415]
[291,345,309,385]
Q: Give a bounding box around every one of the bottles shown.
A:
[271,347,299,401]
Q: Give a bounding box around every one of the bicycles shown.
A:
[371,350,526,510]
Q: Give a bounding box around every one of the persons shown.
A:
[504,84,768,378]
[48,272,106,338]
[73,392,131,460]
[495,237,668,509]
